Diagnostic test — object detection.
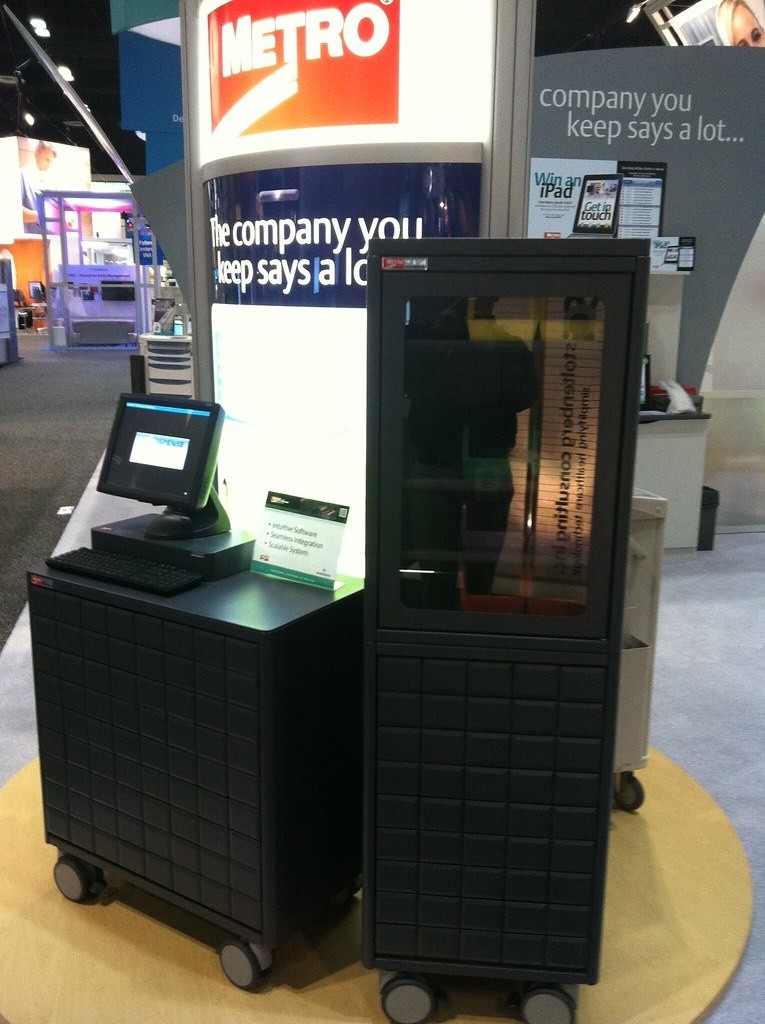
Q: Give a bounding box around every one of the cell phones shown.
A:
[664,246,679,263]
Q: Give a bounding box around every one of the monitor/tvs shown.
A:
[97,392,232,540]
[27,280,43,298]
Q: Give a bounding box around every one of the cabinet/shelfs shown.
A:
[632,270,712,553]
[611,485,668,815]
[22,560,367,991]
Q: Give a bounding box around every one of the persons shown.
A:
[408,295,537,597]
[593,184,602,195]
[715,0,765,47]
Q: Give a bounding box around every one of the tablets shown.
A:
[572,174,624,235]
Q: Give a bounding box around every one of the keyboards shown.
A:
[45,546,206,597]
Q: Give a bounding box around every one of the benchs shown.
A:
[57,319,135,347]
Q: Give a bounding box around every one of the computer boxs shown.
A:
[91,513,255,582]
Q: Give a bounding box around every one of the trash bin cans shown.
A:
[697,484,719,552]
